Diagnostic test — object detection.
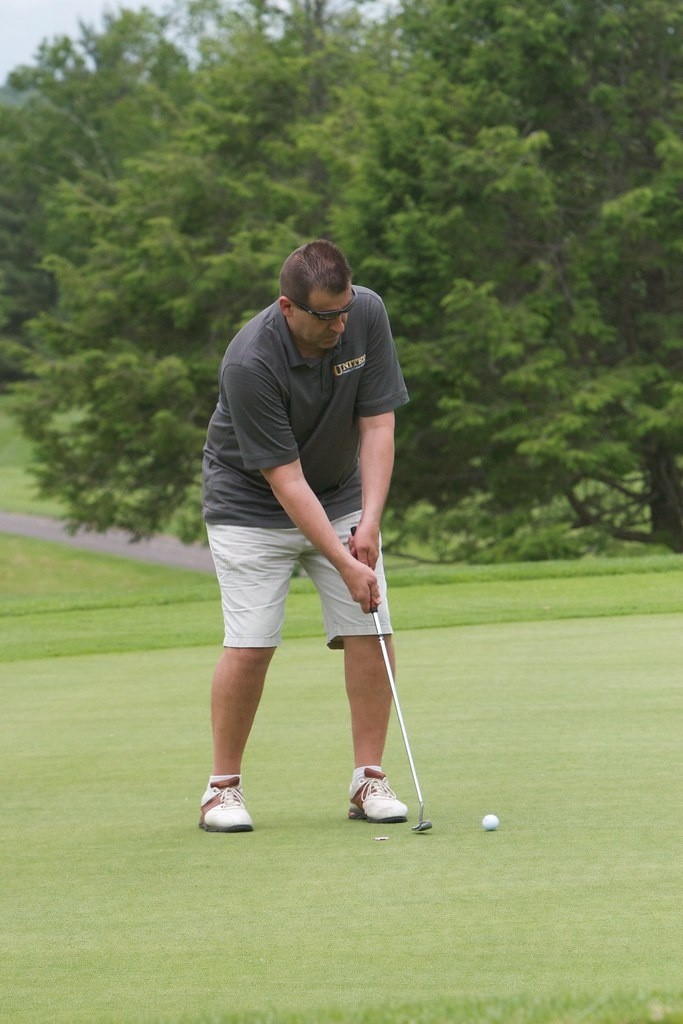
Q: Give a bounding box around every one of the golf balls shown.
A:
[482,814,500,831]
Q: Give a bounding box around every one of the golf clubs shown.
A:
[350,526,433,832]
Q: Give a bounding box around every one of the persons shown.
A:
[200,241,410,831]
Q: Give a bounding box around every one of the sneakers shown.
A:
[349,767,409,824]
[199,777,255,833]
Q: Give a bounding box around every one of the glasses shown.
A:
[281,288,358,320]
